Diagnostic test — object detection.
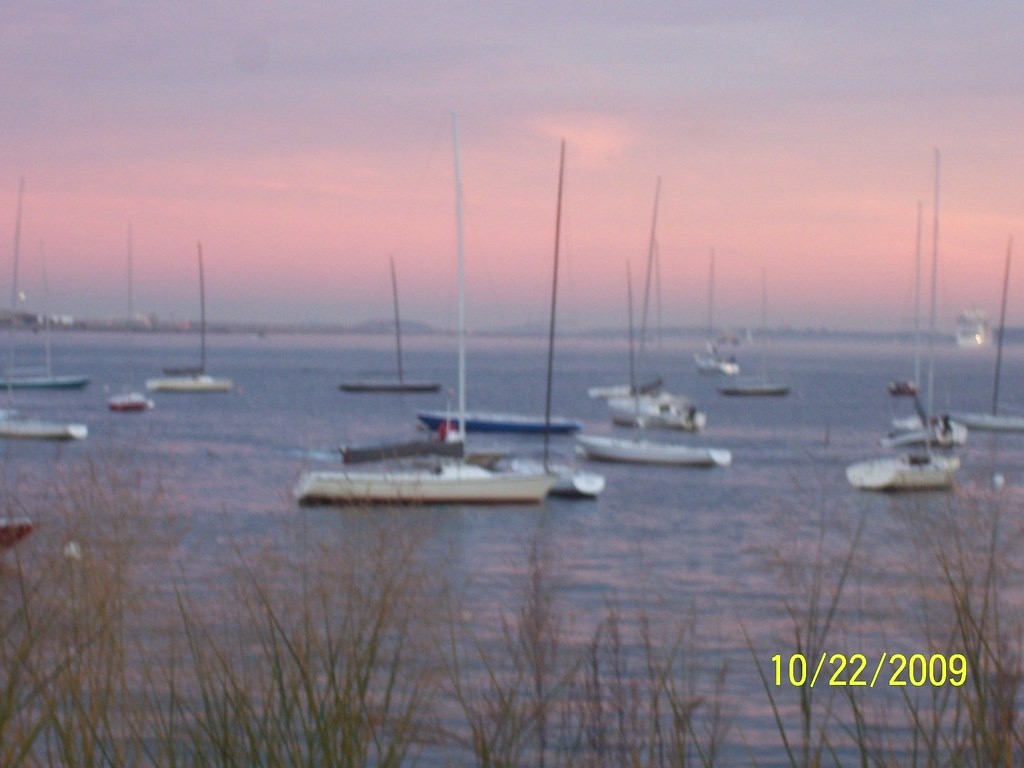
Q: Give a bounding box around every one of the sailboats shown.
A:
[844,145,1023,492]
[0,242,93,394]
[141,242,235,393]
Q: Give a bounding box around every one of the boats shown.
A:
[108,392,148,412]
[1,410,90,443]
[691,352,740,377]
[718,383,792,398]
[300,109,735,509]
